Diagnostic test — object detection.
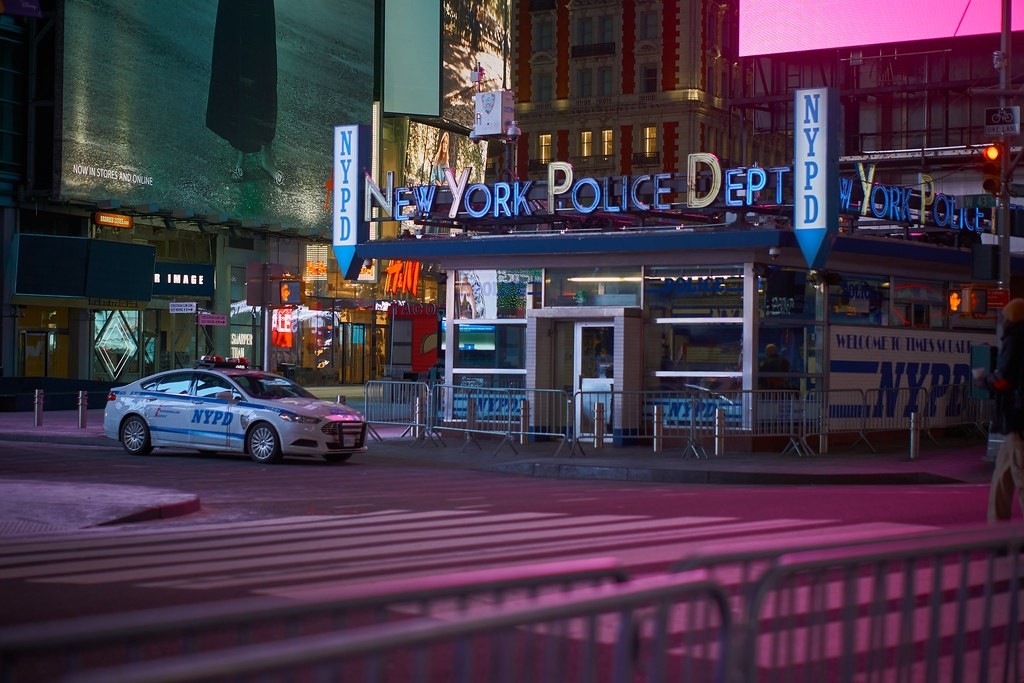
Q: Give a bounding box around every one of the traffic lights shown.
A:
[983,142,1003,196]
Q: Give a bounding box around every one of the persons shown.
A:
[758,343,793,398]
[206,0,285,183]
[983,299,1024,522]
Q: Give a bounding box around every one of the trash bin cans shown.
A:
[277,363,296,383]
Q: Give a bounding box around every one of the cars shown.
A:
[102,357,369,464]
